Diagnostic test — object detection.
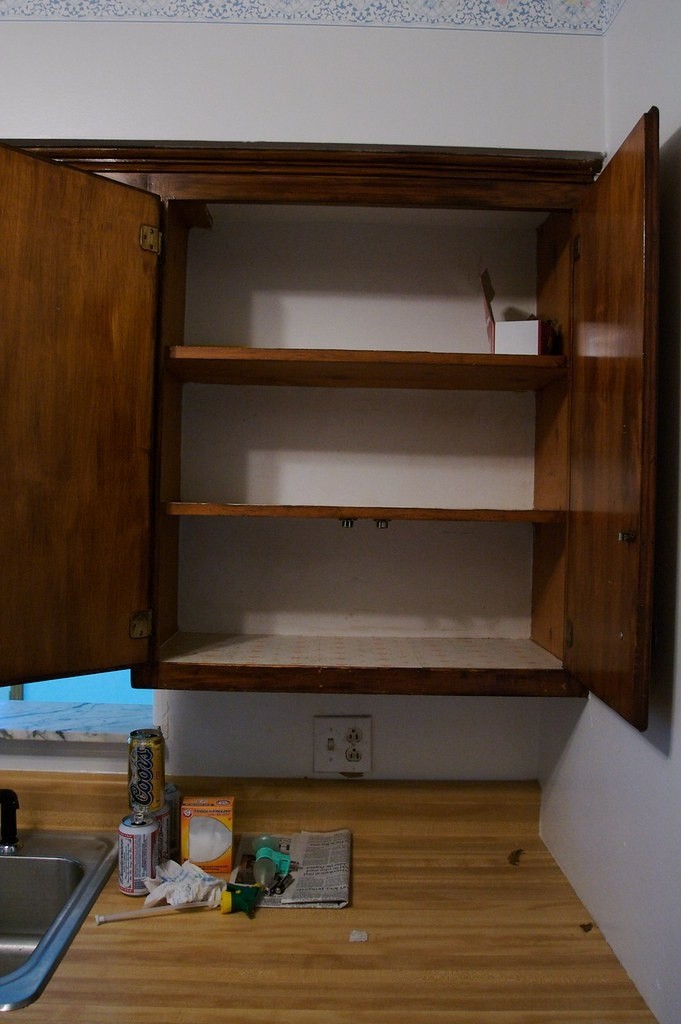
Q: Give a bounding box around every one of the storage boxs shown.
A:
[181,796,233,873]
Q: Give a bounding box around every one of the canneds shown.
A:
[118,729,183,898]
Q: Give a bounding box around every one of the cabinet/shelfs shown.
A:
[0,105,664,734]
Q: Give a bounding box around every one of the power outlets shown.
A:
[313,715,373,773]
